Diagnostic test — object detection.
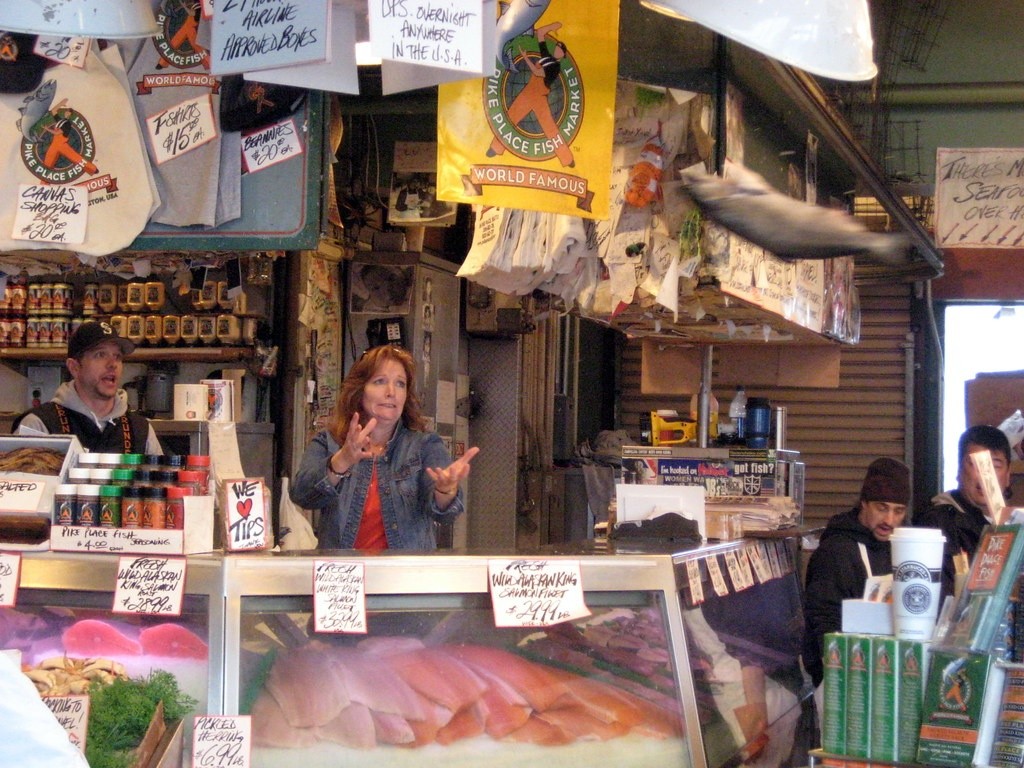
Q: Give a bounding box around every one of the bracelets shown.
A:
[435,485,456,496]
[327,455,354,478]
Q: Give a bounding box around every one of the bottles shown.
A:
[0,279,258,348]
[55,451,210,529]
[729,384,747,445]
[691,377,718,445]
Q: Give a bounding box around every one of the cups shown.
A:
[657,409,678,447]
[890,527,946,640]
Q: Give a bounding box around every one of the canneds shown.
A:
[54,451,209,529]
[0,281,99,349]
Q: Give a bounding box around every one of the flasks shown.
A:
[771,406,788,450]
[746,397,771,449]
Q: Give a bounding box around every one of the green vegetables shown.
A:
[79,669,198,768]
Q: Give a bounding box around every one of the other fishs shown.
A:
[680,170,913,267]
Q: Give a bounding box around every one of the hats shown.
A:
[68,321,135,358]
[861,458,911,505]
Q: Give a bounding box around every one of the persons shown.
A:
[911,425,1013,622]
[351,264,396,312]
[804,457,911,728]
[423,305,433,329]
[423,334,432,359]
[677,538,820,768]
[395,176,452,217]
[423,276,433,302]
[11,321,164,457]
[289,344,478,645]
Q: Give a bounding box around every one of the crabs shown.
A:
[21,655,128,697]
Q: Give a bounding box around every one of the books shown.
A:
[822,523,1024,768]
[945,526,1024,654]
